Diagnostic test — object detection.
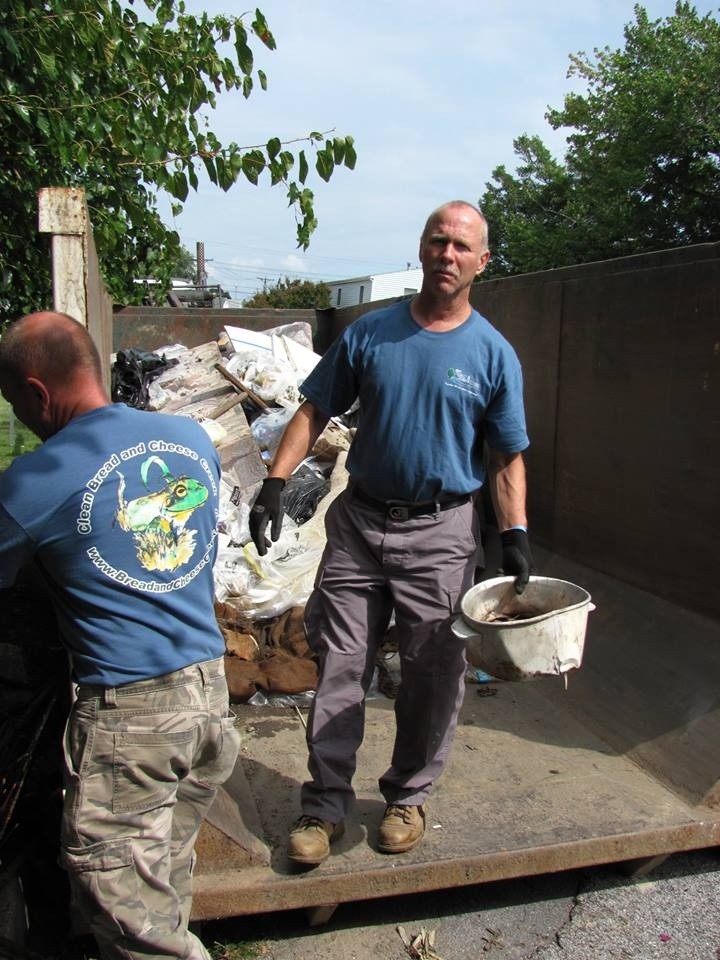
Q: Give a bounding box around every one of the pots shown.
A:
[452,574,596,682]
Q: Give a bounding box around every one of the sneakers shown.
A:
[287,814,344,864]
[376,803,427,852]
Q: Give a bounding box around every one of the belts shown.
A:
[345,483,469,522]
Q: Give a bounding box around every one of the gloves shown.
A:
[499,529,535,594]
[249,477,286,555]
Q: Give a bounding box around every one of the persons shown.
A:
[249,200,533,863]
[0,310,237,960]
